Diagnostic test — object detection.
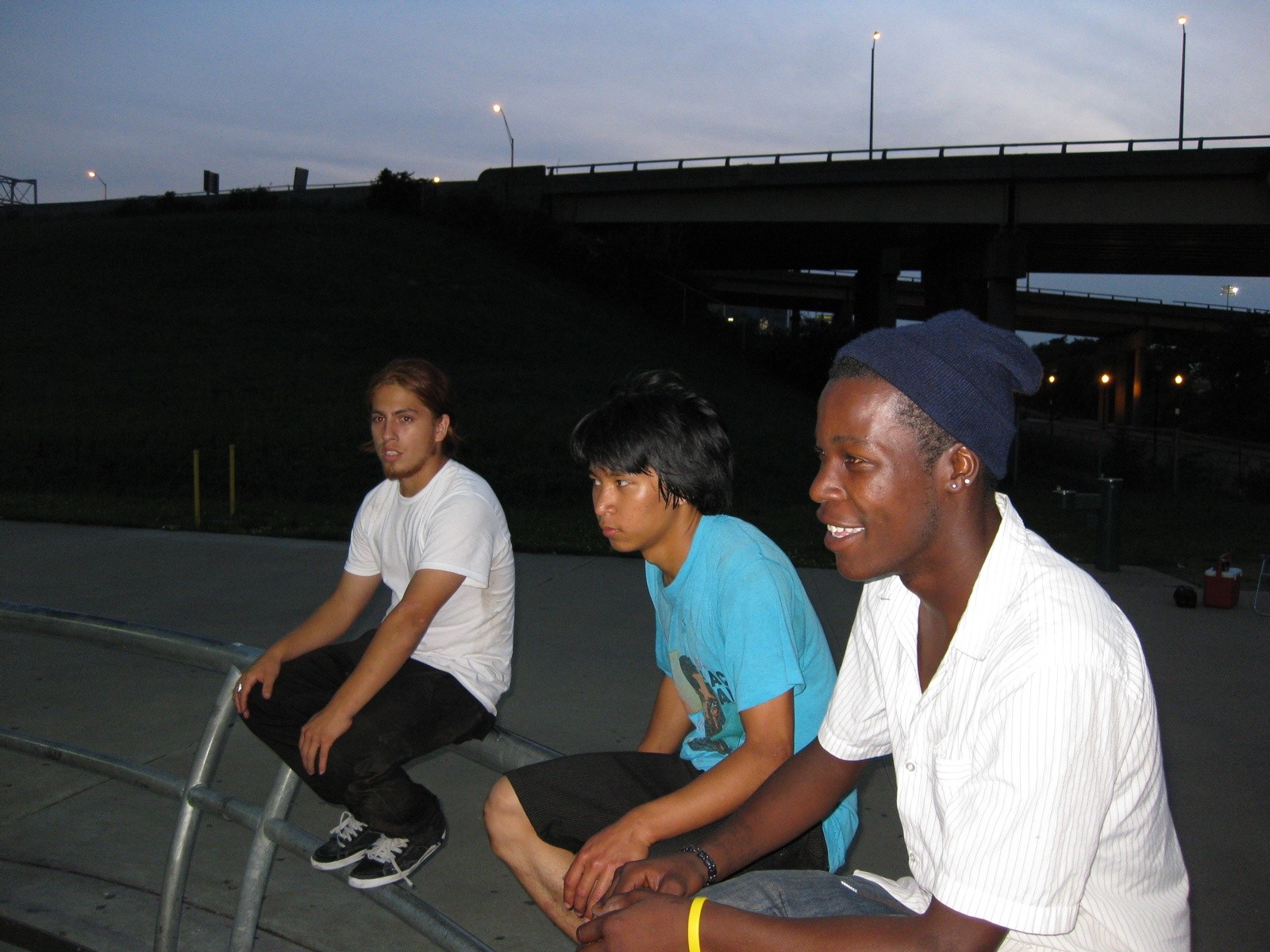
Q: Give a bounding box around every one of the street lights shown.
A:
[1177,16,1187,148]
[88,172,107,201]
[493,103,514,167]
[869,31,880,159]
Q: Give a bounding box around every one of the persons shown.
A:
[232,356,516,889]
[574,312,1192,952]
[483,366,859,947]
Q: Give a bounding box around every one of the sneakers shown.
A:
[348,827,447,890]
[309,811,390,871]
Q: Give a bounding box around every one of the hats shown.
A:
[833,305,1042,480]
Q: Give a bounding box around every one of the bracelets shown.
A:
[687,896,710,952]
[679,845,717,886]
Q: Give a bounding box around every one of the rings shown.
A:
[234,682,242,693]
